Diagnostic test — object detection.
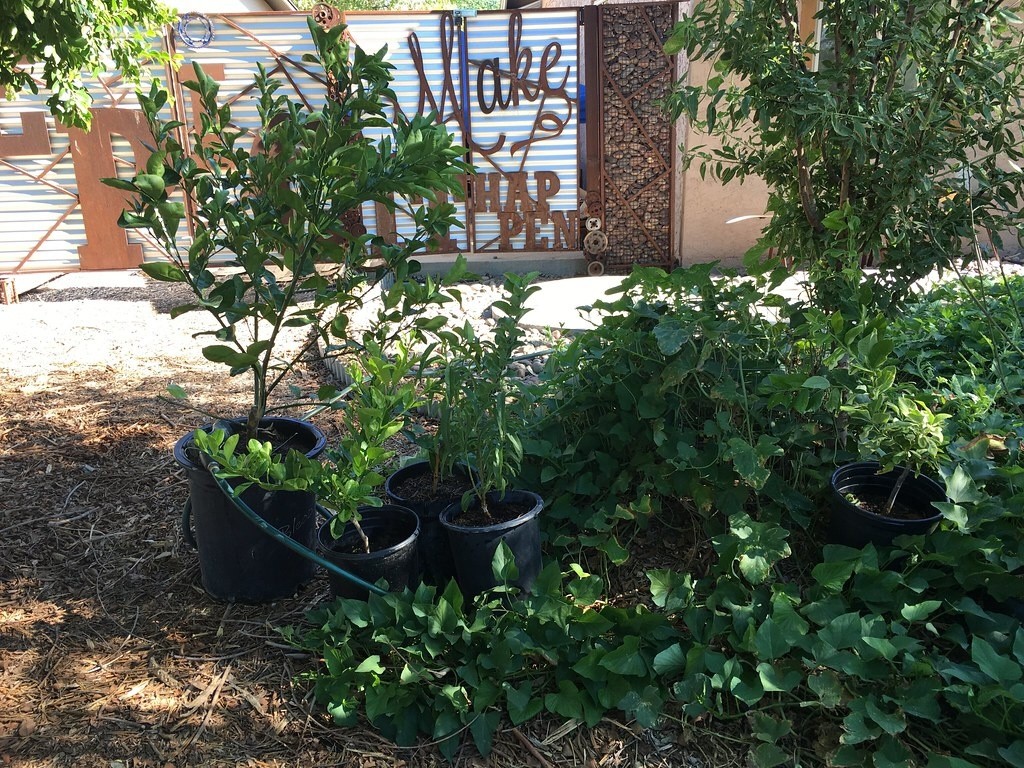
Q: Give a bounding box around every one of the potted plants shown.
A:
[101,26,549,604]
[829,397,951,547]
